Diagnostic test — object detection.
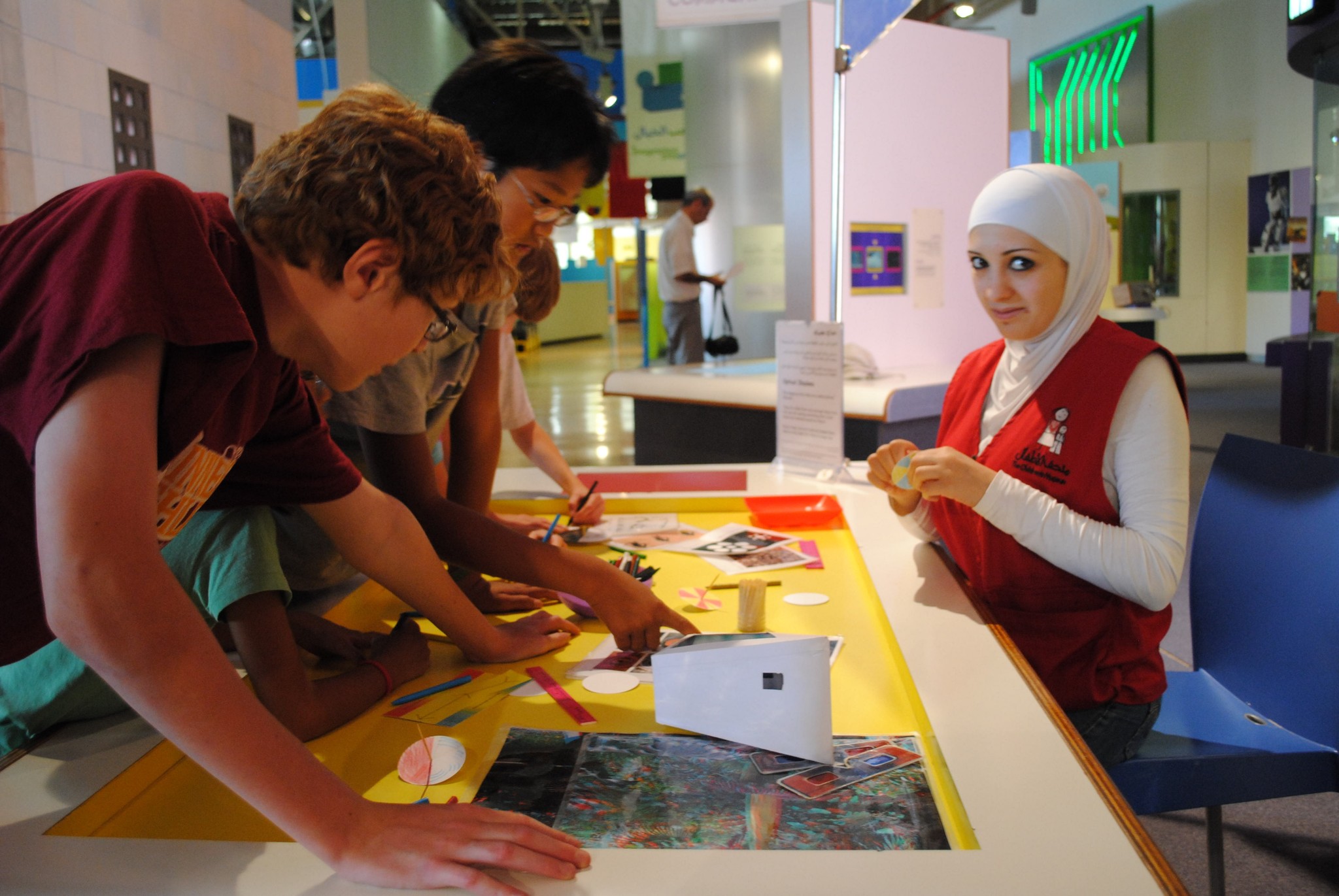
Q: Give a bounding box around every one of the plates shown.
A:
[744,494,843,527]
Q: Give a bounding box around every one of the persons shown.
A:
[0,37,703,896]
[867,163,1191,767]
[1265,174,1286,252]
[658,190,725,366]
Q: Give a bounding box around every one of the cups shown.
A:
[738,579,767,632]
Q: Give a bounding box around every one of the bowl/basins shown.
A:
[557,566,653,619]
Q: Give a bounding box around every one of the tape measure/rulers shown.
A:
[525,666,597,726]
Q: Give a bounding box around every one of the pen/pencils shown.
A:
[392,675,471,706]
[706,581,781,590]
[568,480,597,526]
[609,551,660,582]
[608,545,645,559]
[542,514,560,542]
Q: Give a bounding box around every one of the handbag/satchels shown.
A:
[704,336,738,357]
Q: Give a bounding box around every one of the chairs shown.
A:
[1100,432,1339,896]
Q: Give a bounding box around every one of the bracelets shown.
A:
[364,660,393,696]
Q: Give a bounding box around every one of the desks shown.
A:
[603,357,952,466]
[1265,331,1339,453]
[1100,306,1166,340]
[0,458,1182,896]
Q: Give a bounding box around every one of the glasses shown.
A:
[506,169,577,226]
[410,290,460,343]
